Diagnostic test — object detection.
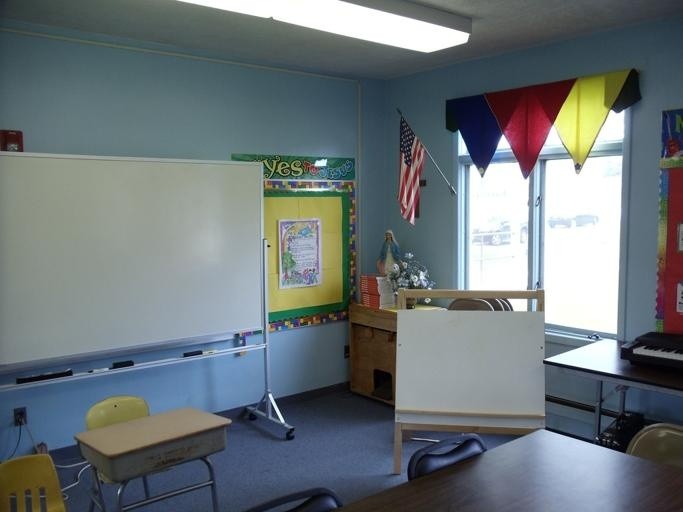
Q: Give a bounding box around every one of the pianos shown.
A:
[620,332,683,375]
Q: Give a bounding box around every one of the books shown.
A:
[358,274,396,309]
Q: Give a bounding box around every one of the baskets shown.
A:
[598,411,643,452]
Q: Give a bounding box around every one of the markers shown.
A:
[203,348,218,356]
[87,366,110,375]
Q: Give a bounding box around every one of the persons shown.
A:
[377,228,402,275]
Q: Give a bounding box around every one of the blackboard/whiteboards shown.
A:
[0,149,267,375]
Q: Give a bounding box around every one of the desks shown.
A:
[311,427,683,512]
[72,407,233,512]
[542,336,683,437]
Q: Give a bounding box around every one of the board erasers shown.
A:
[111,358,135,369]
[182,348,203,357]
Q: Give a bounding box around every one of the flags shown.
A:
[397,115,428,230]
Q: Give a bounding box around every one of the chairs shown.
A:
[1,453,65,512]
[243,486,343,511]
[624,422,683,469]
[406,433,487,482]
[85,396,153,511]
